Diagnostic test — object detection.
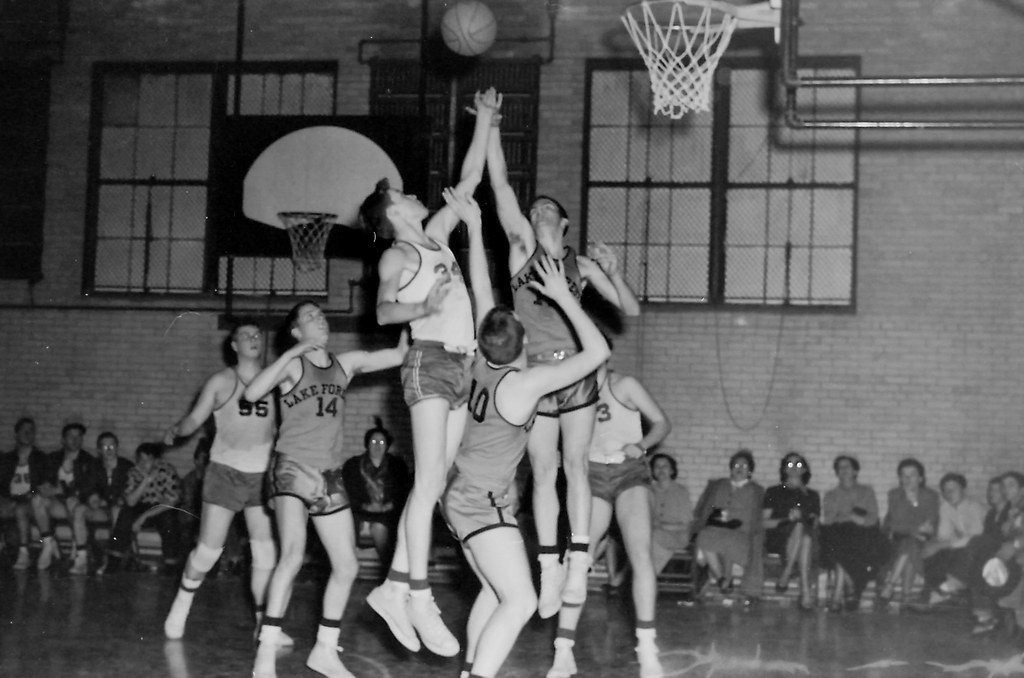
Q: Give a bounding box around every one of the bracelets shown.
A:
[636,443,647,454]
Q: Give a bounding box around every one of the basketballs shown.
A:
[440,0,499,57]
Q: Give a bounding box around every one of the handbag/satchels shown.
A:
[707,507,743,530]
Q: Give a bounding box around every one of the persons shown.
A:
[466,90,640,619]
[610,454,692,598]
[441,186,611,677]
[545,341,676,674]
[244,303,413,678]
[698,450,1024,635]
[163,322,298,645]
[344,429,411,569]
[363,89,503,650]
[0,418,219,579]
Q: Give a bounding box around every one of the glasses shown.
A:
[732,463,750,469]
[784,462,804,469]
[370,439,387,445]
[100,444,117,451]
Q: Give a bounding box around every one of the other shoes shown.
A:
[160,561,179,576]
[164,598,190,639]
[104,554,122,573]
[253,623,294,645]
[37,544,56,569]
[701,570,1007,635]
[97,560,108,575]
[206,559,219,578]
[14,552,30,570]
[69,554,88,575]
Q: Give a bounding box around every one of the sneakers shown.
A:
[561,556,591,604]
[633,641,662,678]
[366,585,420,652]
[538,564,568,619]
[547,647,577,678]
[406,588,460,657]
[306,643,355,678]
[252,642,277,678]
